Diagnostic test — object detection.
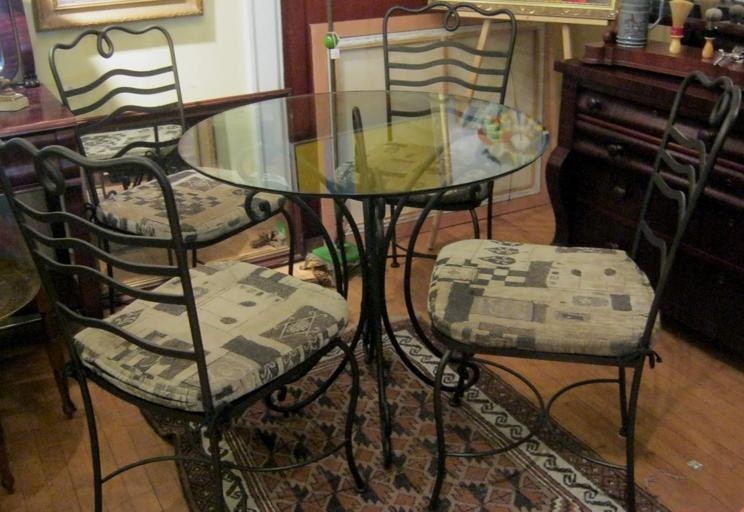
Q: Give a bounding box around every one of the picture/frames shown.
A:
[31,0,203,33]
[309,12,551,245]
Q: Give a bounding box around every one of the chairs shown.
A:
[427,70,744,512]
[47,24,295,316]
[335,1,518,268]
[0,137,369,512]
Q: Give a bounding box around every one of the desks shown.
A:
[177,90,550,470]
[0,1,104,492]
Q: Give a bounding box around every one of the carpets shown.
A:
[138,317,673,512]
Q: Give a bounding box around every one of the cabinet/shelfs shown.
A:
[545,31,743,372]
